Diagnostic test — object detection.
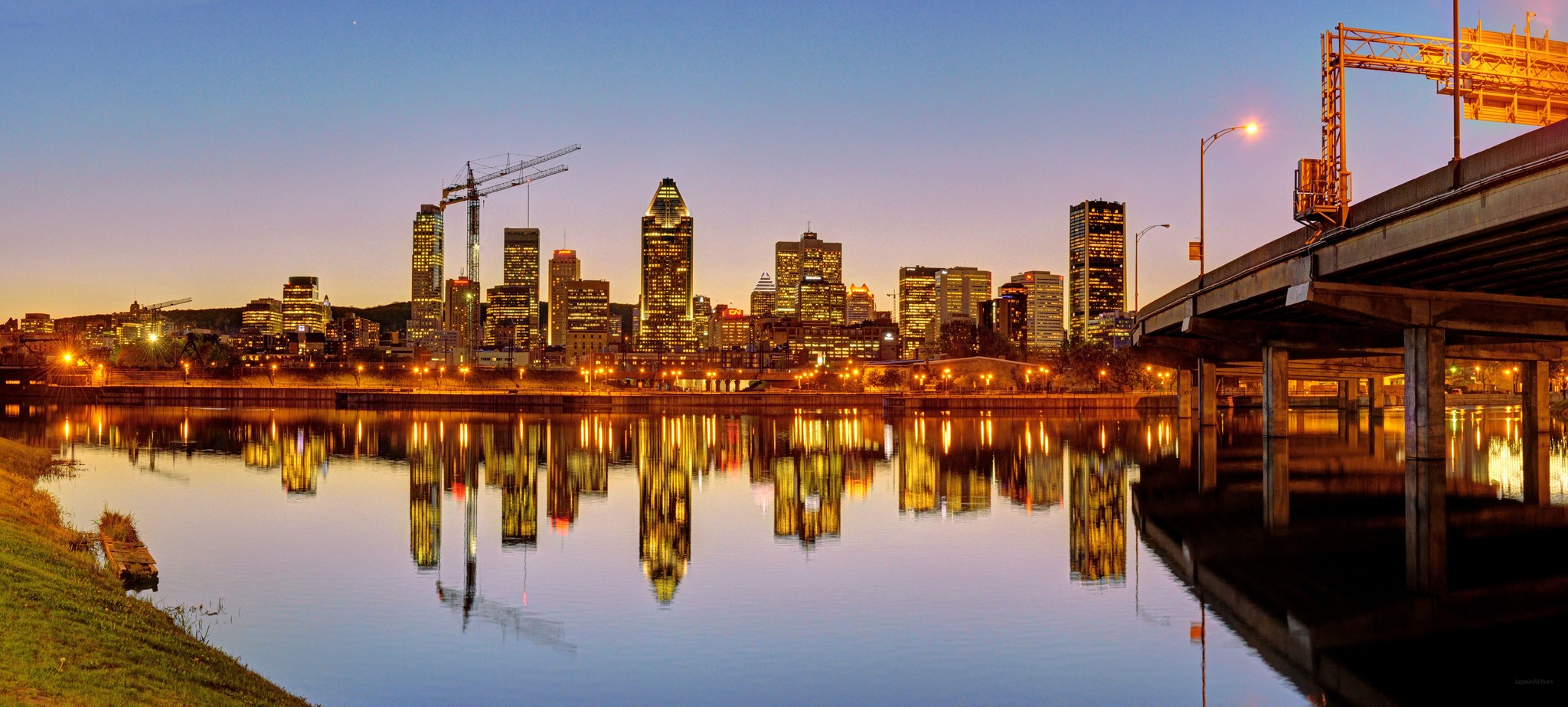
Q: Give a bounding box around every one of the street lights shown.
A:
[439,367,444,388]
[1158,372,1169,394]
[803,372,813,391]
[981,374,992,391]
[519,369,524,386]
[795,375,803,390]
[1098,371,1105,390]
[1134,223,1171,328]
[640,369,644,382]
[1199,120,1261,279]
[942,374,951,391]
[599,367,613,394]
[99,364,103,386]
[1512,367,1518,394]
[662,373,666,387]
[260,364,262,372]
[839,373,850,392]
[461,367,469,393]
[707,372,716,394]
[272,365,276,386]
[359,366,362,387]
[1040,367,1049,391]
[185,364,188,384]
[914,374,926,392]
[1476,366,1480,393]
[414,368,428,392]
[672,371,681,393]
[594,370,598,388]
[1024,370,1031,391]
[581,370,589,394]
[1147,365,1152,391]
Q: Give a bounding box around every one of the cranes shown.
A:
[136,296,194,314]
[887,289,900,323]
[438,142,581,370]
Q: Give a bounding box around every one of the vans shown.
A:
[1451,388,1463,396]
